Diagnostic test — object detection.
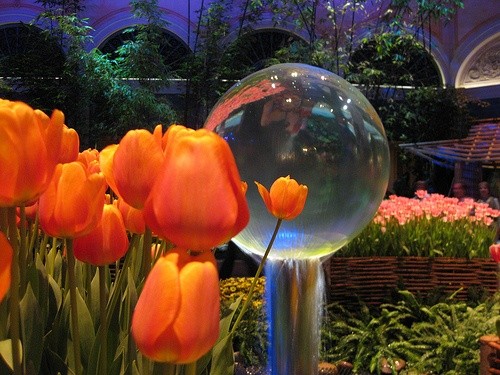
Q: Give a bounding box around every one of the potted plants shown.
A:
[227,281,500,375]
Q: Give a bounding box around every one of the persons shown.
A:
[258,89,315,131]
[413,179,432,200]
[451,180,466,198]
[476,181,500,210]
